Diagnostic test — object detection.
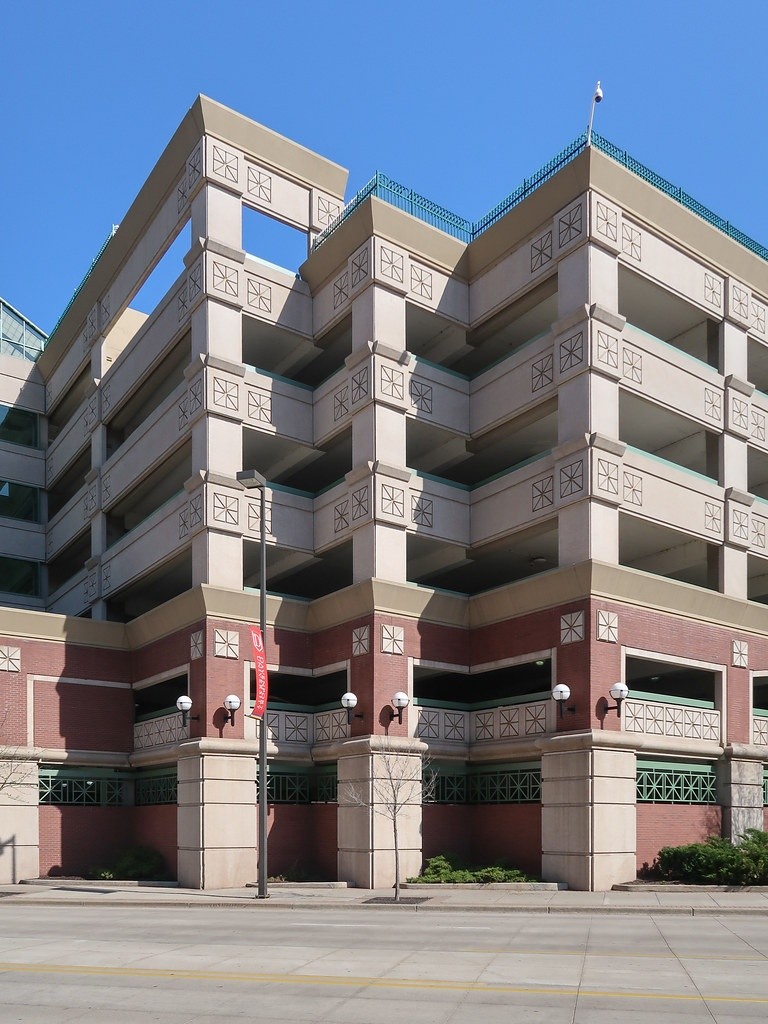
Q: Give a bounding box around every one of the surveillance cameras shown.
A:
[595,88,603,103]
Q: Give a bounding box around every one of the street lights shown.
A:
[238,469,272,898]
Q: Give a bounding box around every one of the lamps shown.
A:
[223,694,241,727]
[342,692,364,725]
[551,684,576,718]
[389,692,411,724]
[177,696,200,727]
[605,683,629,717]
